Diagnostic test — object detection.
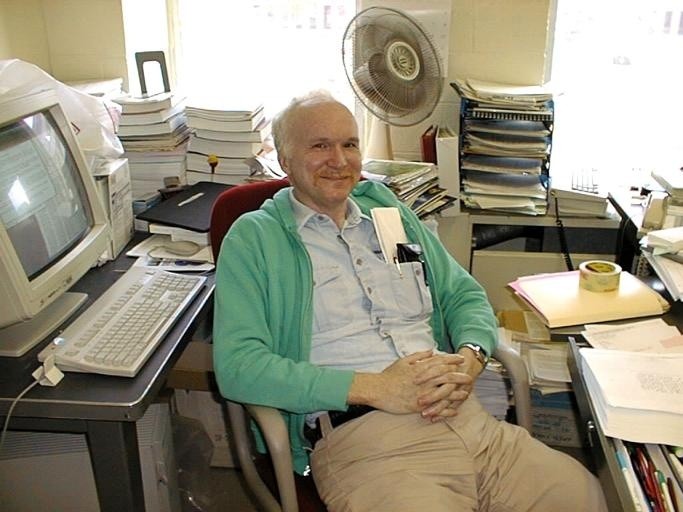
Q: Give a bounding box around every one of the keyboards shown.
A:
[37,265,208,378]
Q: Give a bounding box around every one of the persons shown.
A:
[210,95,608,512]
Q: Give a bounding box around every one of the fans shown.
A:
[338,5,446,161]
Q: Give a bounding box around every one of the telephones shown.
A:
[641,191,683,231]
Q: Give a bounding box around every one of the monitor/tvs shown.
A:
[0,88,113,357]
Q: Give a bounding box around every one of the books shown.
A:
[474,309,585,450]
[108,89,274,192]
[357,78,553,219]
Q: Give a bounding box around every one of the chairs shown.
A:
[203,178,533,512]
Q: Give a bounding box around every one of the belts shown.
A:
[303,402,379,441]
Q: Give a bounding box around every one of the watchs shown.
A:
[459,343,488,369]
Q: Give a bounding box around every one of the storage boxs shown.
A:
[526,386,582,452]
[172,389,235,468]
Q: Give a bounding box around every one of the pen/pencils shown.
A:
[177,193,205,207]
[422,260,429,286]
[394,257,404,280]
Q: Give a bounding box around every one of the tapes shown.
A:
[579,260,623,292]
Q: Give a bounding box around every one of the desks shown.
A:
[431,207,622,294]
[0,216,220,512]
[567,192,682,510]
